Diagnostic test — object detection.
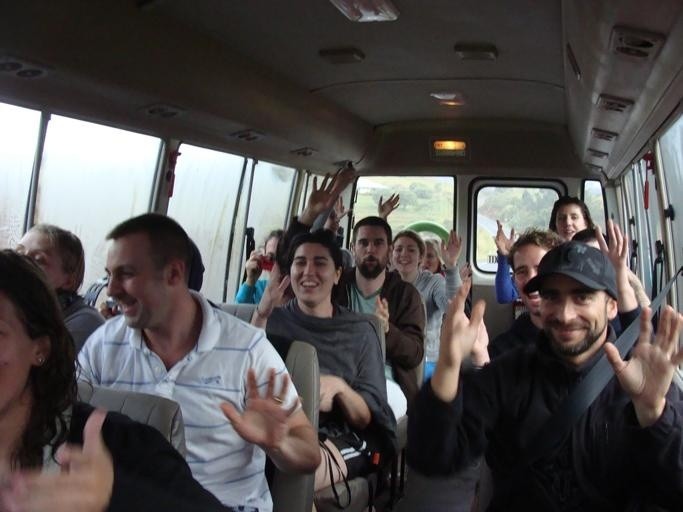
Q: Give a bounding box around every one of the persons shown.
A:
[1,168,683,510]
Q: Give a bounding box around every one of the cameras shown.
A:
[258,255,274,271]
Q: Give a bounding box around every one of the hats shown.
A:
[524,240,618,301]
